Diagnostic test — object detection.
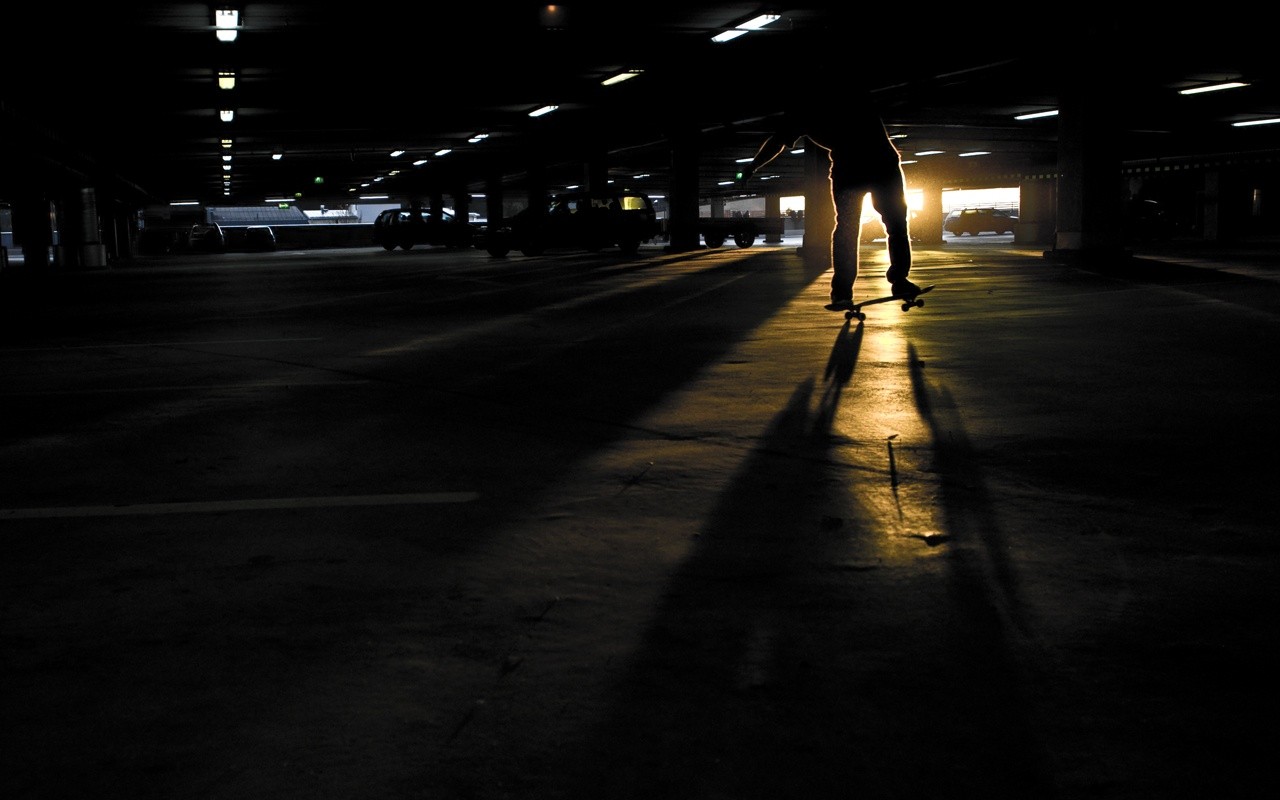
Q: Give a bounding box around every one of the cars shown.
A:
[944,208,1020,237]
[1068,205,1223,283]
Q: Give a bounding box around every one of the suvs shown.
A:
[491,187,656,260]
[373,208,494,253]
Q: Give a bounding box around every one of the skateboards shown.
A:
[824,284,936,322]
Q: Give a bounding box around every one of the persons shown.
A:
[802,31,922,307]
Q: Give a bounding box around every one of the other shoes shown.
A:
[891,280,920,295]
[830,288,853,304]
